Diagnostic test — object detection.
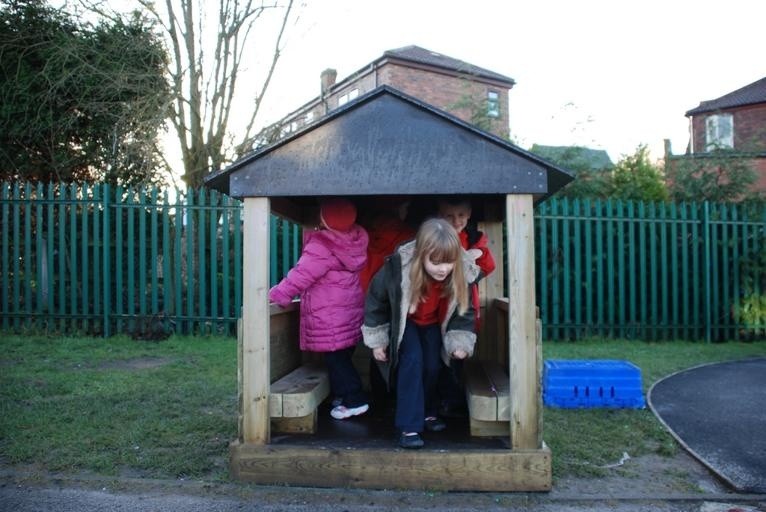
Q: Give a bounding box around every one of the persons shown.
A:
[268,198,370,419]
[361,218,484,449]
[439,196,495,419]
[351,196,416,393]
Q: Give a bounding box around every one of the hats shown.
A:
[320,199,358,232]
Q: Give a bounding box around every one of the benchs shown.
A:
[268,363,329,437]
[463,358,511,439]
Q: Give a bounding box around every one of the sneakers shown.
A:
[424,415,446,432]
[331,403,371,419]
[332,397,343,406]
[399,431,424,449]
